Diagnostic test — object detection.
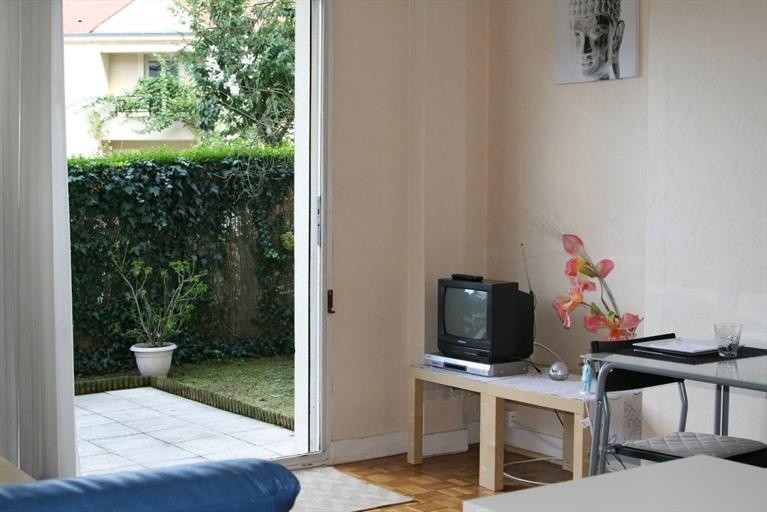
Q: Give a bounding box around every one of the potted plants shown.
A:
[107,240,209,380]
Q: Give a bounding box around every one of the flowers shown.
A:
[551,234,645,341]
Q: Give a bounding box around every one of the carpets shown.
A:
[286,461,418,512]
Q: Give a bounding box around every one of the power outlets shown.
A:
[505,409,518,431]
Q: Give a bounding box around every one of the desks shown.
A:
[407,363,643,493]
[579,346,766,474]
[461,453,766,511]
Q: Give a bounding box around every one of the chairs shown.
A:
[589,332,766,477]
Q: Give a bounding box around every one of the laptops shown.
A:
[632,337,745,361]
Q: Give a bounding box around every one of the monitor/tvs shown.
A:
[437,277,534,365]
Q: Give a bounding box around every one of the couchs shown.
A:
[0,458,301,512]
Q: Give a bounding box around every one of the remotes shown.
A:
[452,273,483,283]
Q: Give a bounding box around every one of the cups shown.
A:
[715,322,742,358]
